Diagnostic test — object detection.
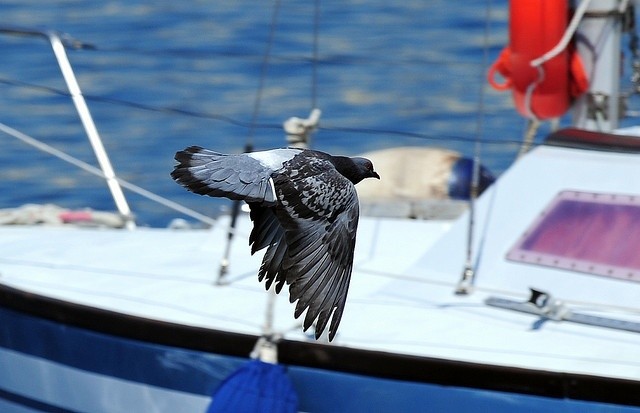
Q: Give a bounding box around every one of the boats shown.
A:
[0,0,640,413]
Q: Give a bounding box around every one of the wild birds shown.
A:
[172,141,380,344]
